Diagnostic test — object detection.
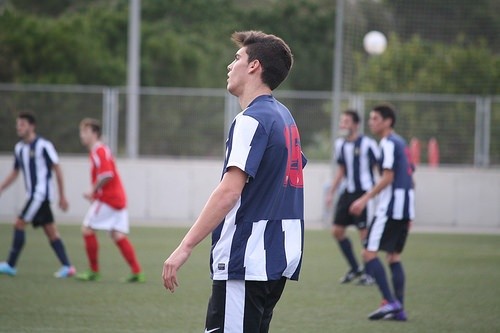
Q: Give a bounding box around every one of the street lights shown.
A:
[362,31,386,102]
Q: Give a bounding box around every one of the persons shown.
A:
[348,104,416,322]
[324,109,384,286]
[162,30,308,332]
[0,112,76,278]
[73,117,144,283]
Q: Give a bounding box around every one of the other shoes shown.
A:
[74,269,101,281]
[120,273,145,283]
[53,266,76,277]
[0,261,17,276]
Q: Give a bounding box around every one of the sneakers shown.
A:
[381,309,407,321]
[341,267,364,284]
[368,300,402,320]
[358,277,376,286]
